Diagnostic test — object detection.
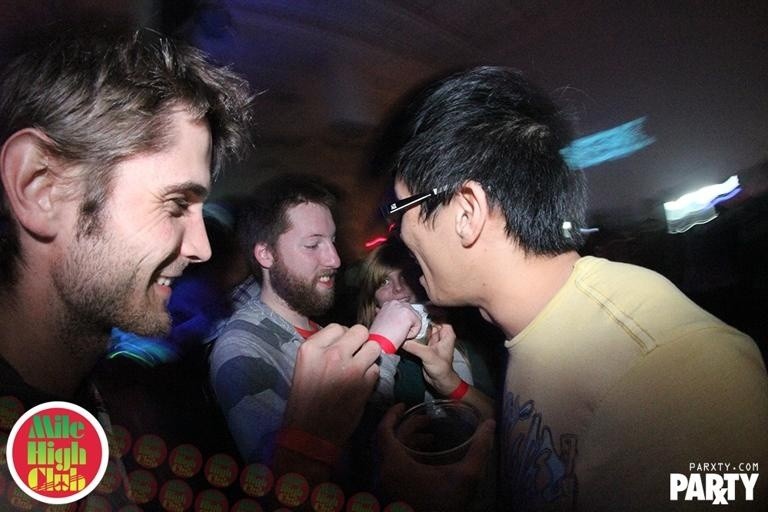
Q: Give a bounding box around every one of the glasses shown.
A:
[379,183,457,222]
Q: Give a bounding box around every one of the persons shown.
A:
[0,12,768,512]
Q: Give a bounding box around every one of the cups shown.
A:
[398,400,482,463]
